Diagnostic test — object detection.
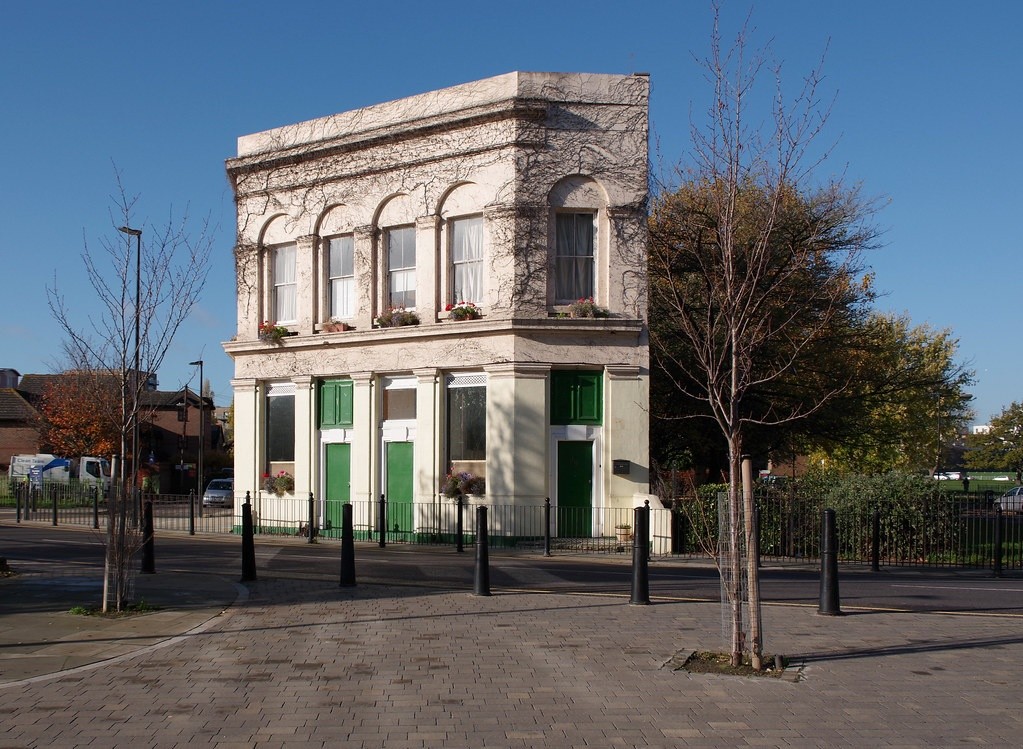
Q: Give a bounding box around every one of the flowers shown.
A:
[568,296,604,318]
[445,301,482,321]
[376,306,419,328]
[262,470,294,497]
[256,321,287,344]
[441,467,487,496]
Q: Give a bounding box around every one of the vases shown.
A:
[464,311,473,320]
[452,314,462,321]
[570,309,592,318]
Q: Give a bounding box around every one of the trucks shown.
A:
[8,454,111,496]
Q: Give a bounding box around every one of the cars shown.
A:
[933,473,949,480]
[992,487,1023,513]
[762,476,798,492]
[221,468,234,480]
[945,472,962,480]
[992,475,1012,481]
[203,479,234,509]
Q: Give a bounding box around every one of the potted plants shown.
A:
[322,320,348,332]
[616,523,632,541]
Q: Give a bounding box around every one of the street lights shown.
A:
[119,226,143,529]
[189,360,203,518]
[933,393,941,480]
